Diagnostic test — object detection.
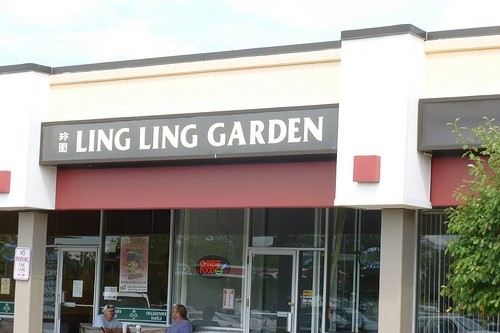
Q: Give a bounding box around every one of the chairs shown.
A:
[81,326,105,333]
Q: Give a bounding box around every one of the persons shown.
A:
[92,304,123,333]
[142,304,193,333]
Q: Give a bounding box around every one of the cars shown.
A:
[282,265,500,333]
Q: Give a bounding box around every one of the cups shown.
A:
[135,324,142,333]
[122,324,127,333]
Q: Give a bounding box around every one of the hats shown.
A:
[102,304,115,312]
